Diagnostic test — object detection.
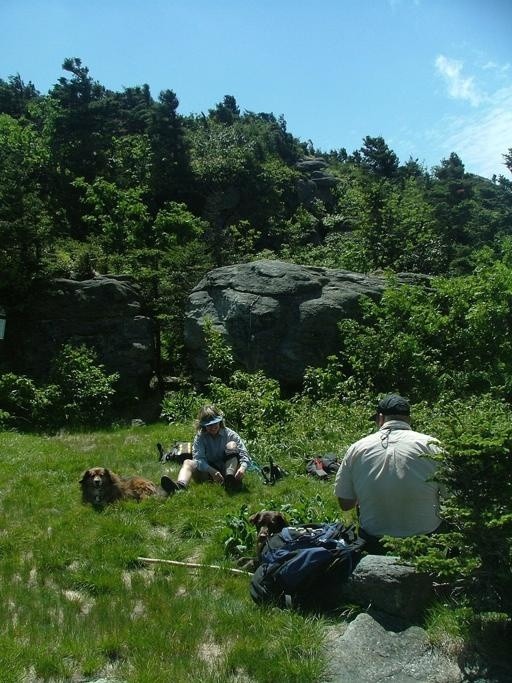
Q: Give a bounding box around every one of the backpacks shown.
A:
[246,523,368,607]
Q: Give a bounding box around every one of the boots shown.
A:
[160,475,188,495]
[224,476,240,496]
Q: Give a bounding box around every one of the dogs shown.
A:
[248,510,290,553]
[78,467,160,513]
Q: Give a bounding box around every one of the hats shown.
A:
[199,415,223,426]
[368,394,410,421]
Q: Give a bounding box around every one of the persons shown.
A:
[332,394,453,554]
[159,403,251,497]
